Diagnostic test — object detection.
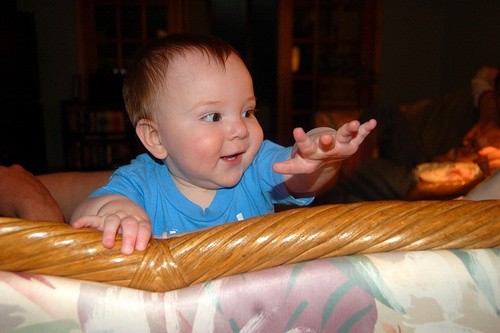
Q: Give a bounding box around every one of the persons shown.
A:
[311,66,500,207]
[70,31,377,254]
[0,164,66,223]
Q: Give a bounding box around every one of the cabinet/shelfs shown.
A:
[244,0,384,148]
